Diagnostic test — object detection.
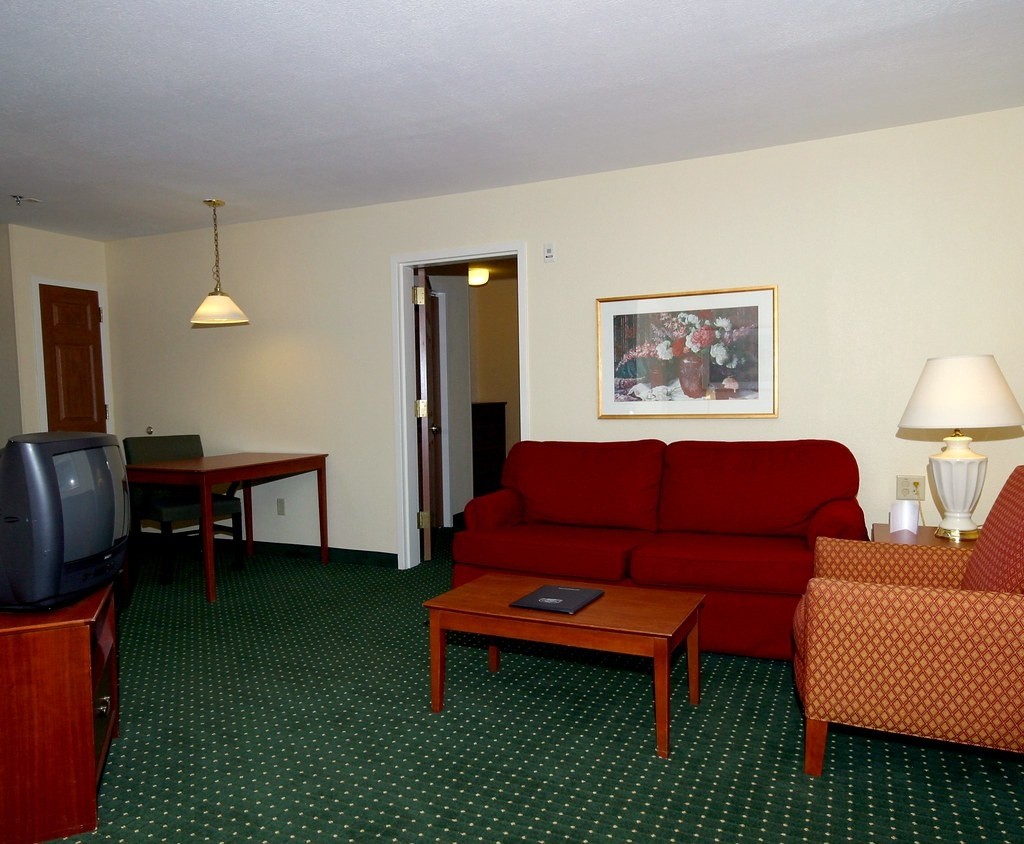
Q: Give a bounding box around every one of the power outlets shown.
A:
[277,498,285,515]
[895,474,926,502]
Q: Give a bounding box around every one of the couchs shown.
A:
[451,439,866,662]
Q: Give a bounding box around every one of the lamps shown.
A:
[899,353,1024,540]
[190,198,250,328]
[468,267,490,285]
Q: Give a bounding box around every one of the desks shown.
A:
[125,452,330,603]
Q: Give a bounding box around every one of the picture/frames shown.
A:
[594,283,778,420]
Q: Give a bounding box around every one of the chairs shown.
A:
[122,434,243,587]
[792,465,1024,778]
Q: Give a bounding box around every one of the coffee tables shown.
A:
[423,574,709,758]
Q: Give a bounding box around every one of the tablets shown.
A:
[509,585,605,615]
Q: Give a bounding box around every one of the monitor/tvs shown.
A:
[0,431,131,614]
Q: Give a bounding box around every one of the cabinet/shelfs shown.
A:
[0,584,119,844]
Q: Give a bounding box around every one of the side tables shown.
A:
[870,520,984,545]
